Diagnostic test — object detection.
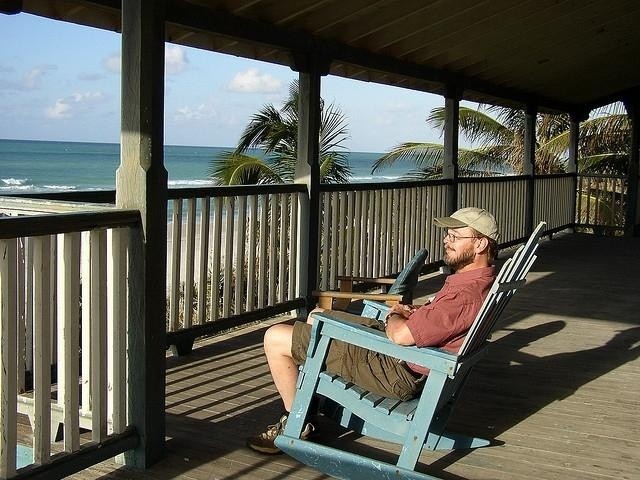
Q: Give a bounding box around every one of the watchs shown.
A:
[385,313,400,324]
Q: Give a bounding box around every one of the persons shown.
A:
[245,206,502,454]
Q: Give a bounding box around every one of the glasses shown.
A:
[442,230,481,243]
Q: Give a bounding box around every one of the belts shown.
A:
[399,360,422,379]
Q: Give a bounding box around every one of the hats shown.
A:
[433,207,500,242]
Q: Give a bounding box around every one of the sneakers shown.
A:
[249,412,315,455]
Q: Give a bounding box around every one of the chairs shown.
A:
[312,248,428,312]
[272,221,547,480]
